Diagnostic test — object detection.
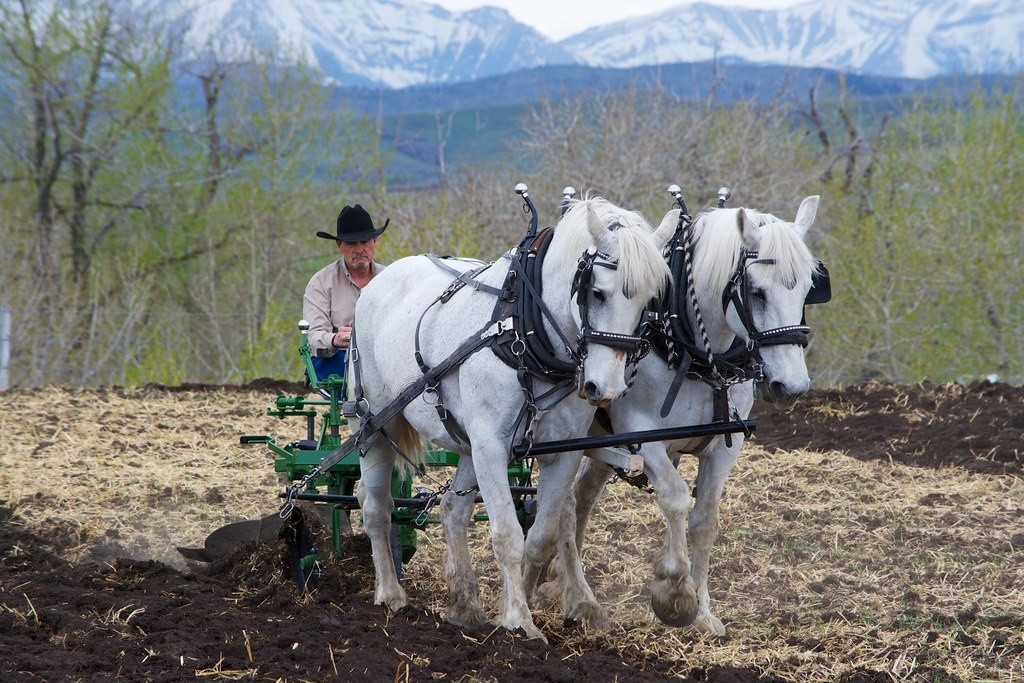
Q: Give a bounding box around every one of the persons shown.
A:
[303,204,390,402]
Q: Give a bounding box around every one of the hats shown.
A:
[316,204,390,242]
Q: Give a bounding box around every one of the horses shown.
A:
[340,183,826,651]
